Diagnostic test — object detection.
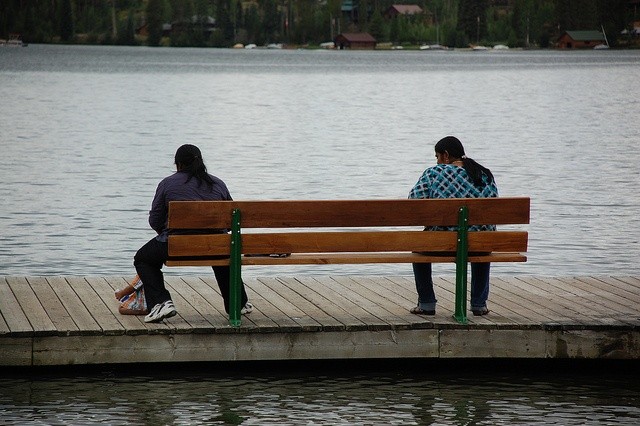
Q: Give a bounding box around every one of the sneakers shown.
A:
[241,303,253,315]
[144,300,176,324]
[473,309,489,316]
[410,306,435,315]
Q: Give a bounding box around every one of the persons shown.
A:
[133,145,254,324]
[408,136,499,316]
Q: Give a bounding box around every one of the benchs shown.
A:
[163,197,529,328]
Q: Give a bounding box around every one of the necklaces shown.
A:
[452,160,462,163]
